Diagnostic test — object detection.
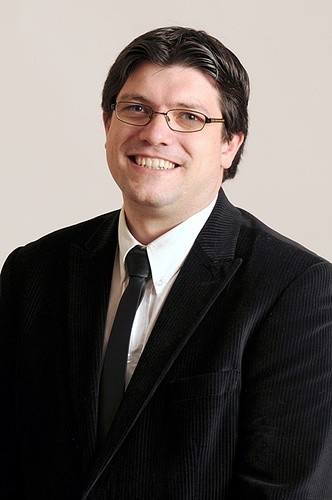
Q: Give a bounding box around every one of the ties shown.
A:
[97,245,152,446]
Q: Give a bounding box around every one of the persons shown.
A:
[1,26,331,500]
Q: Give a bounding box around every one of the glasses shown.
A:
[110,102,226,132]
